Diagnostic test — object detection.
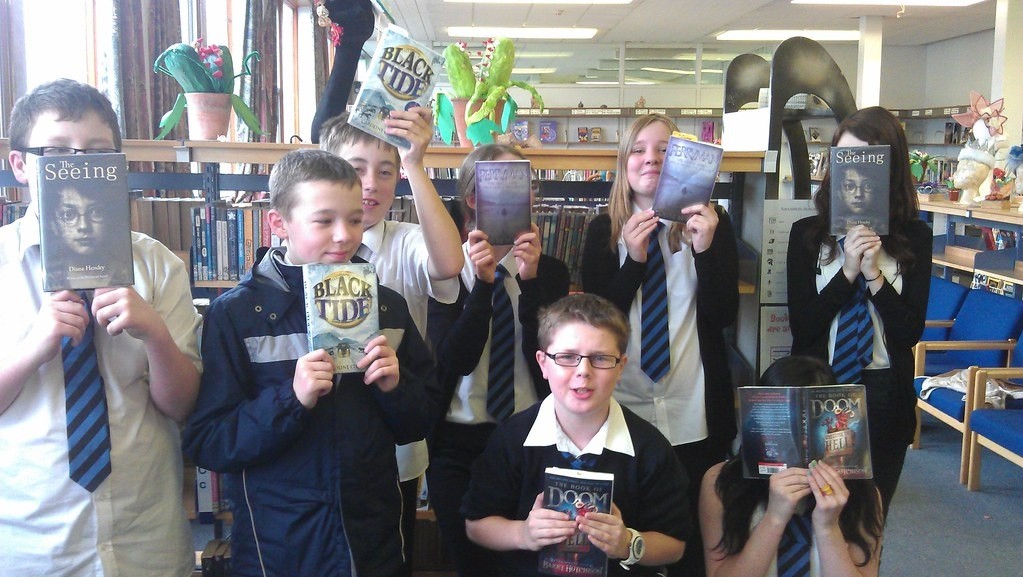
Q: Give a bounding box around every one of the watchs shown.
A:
[619,528,645,570]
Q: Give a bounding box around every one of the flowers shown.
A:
[150,37,272,139]
[907,147,947,181]
[436,38,546,149]
[953,88,1007,138]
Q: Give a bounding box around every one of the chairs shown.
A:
[910,275,969,356]
[909,330,1023,484]
[910,288,1023,450]
[967,369,1023,492]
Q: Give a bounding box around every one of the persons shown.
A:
[423,114,738,577]
[698,355,883,577]
[786,107,934,521]
[0,77,204,577]
[49,185,125,288]
[952,118,1006,206]
[182,107,464,577]
[837,164,879,225]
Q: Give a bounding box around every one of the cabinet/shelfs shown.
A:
[913,198,1023,301]
[116,36,855,539]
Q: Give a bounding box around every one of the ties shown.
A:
[641,221,672,383]
[488,265,516,427]
[776,500,816,577]
[831,237,876,383]
[59,284,112,493]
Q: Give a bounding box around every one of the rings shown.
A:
[821,486,833,496]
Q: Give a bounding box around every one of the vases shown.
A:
[449,98,514,148]
[183,92,232,141]
[911,163,927,185]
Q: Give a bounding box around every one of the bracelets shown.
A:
[866,270,882,282]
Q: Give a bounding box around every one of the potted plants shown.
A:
[945,181,961,201]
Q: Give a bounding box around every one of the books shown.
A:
[652,137,723,222]
[302,263,379,374]
[385,121,620,282]
[348,28,445,149]
[36,152,135,293]
[537,466,614,577]
[809,127,890,237]
[735,384,873,479]
[0,197,287,515]
[926,122,973,184]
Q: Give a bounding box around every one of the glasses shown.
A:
[541,351,620,369]
[12,144,119,156]
